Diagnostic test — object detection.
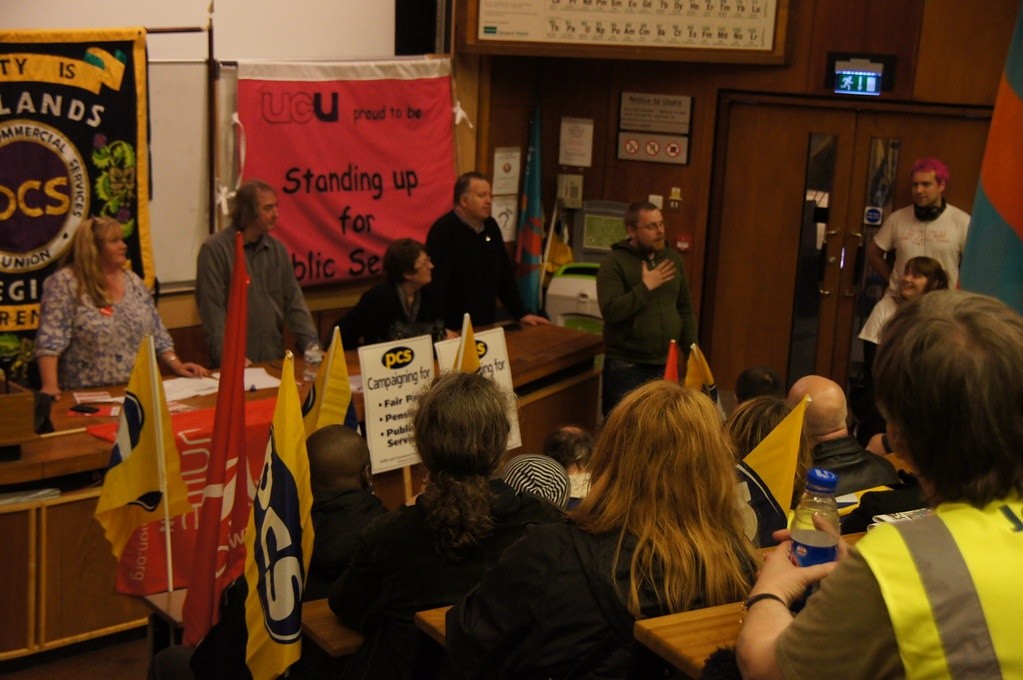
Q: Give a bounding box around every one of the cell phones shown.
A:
[70,404,99,413]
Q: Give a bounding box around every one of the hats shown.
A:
[501,453,570,509]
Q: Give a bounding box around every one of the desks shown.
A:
[0,314,875,680]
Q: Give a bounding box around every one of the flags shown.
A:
[962,0,1023,317]
[663,340,727,424]
[94,236,360,680]
[729,400,807,548]
[453,316,484,376]
[511,110,571,316]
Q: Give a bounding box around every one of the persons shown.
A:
[333,374,564,680]
[731,394,808,512]
[194,185,327,367]
[33,217,205,397]
[786,374,897,534]
[443,381,748,680]
[862,162,971,452]
[302,425,386,602]
[736,290,1023,680]
[594,200,693,420]
[333,172,550,350]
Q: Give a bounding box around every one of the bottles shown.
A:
[303,335,323,382]
[787,469,840,619]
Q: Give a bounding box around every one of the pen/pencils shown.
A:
[40,428,87,438]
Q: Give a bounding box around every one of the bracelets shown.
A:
[311,344,319,351]
[165,356,178,366]
[737,593,786,624]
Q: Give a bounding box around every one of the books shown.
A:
[209,368,281,390]
[159,374,220,401]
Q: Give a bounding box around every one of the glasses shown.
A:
[636,221,665,229]
[415,257,431,270]
